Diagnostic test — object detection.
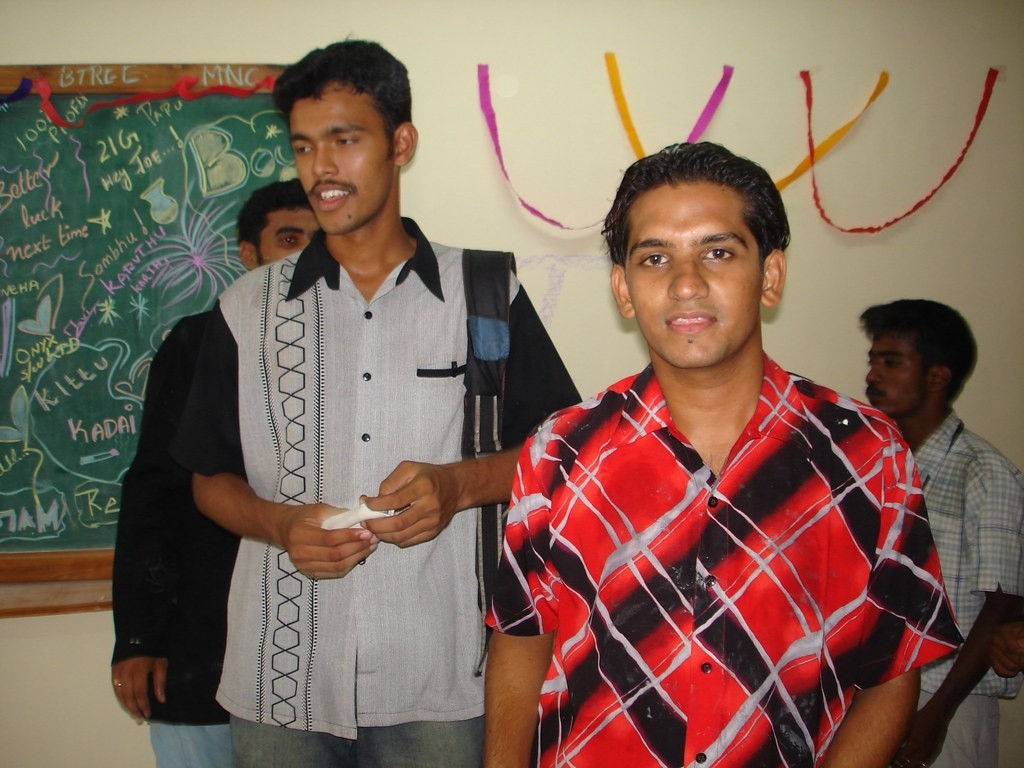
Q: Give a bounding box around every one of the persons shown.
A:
[483,141,966,768]
[858,299,1024,768]
[167,38,583,768]
[111,176,321,768]
[988,620,1024,679]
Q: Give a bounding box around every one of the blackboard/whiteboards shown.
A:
[0,63,313,585]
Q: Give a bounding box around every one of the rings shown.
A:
[113,679,121,687]
